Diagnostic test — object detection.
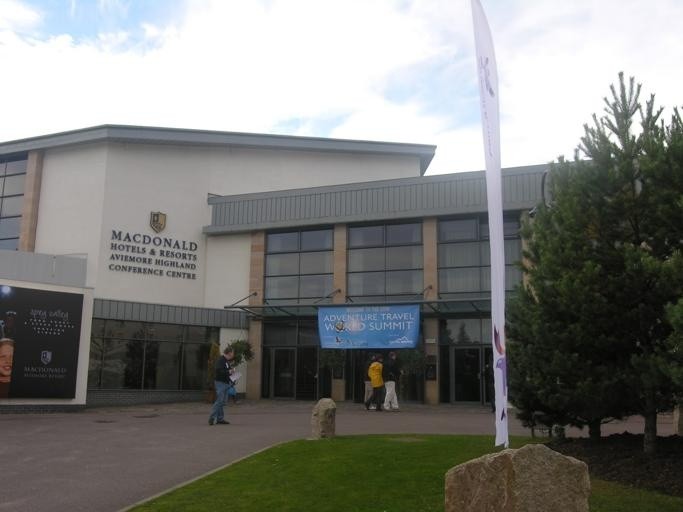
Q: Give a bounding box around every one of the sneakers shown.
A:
[207,417,229,425]
[365,402,400,412]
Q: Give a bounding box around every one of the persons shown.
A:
[363,354,386,411]
[207,346,236,425]
[0,337,15,383]
[361,352,377,411]
[382,351,404,412]
[0,310,16,339]
